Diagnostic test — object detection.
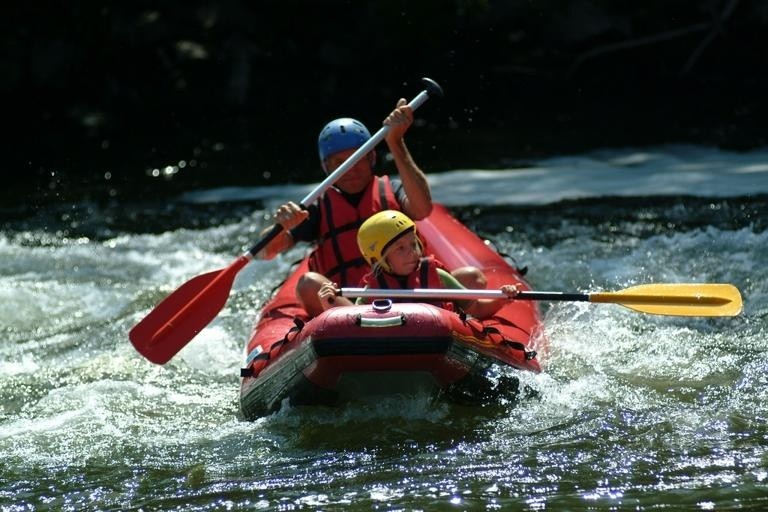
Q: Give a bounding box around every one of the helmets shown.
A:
[318,117,375,187]
[356,208,425,274]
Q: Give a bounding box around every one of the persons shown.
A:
[315,210,522,323]
[259,98,487,318]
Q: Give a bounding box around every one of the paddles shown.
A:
[129,79,445,365]
[325,284,742,316]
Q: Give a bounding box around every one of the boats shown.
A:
[235,201,550,426]
